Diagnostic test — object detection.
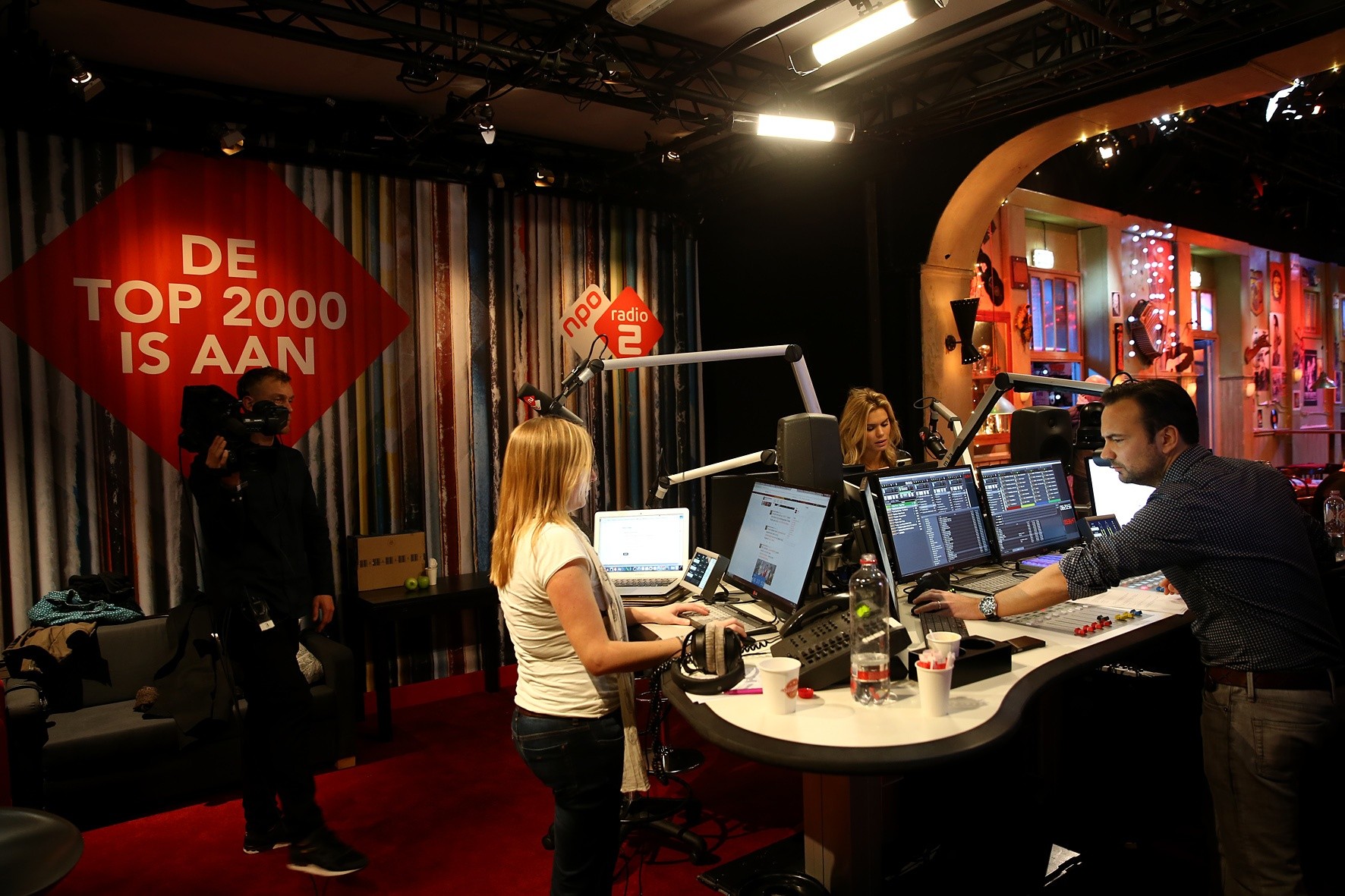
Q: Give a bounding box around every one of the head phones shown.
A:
[670,627,745,695]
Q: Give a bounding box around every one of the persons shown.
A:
[188,366,373,878]
[832,380,912,484]
[1250,268,1282,364]
[911,377,1345,896]
[488,416,750,896]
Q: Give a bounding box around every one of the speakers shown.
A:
[1010,405,1075,477]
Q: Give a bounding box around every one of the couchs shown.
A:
[4,605,362,831]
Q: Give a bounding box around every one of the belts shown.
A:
[1201,662,1345,694]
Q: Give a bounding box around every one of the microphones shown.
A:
[516,381,584,428]
[918,426,948,460]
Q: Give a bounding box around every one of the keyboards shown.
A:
[677,602,777,637]
[920,615,969,649]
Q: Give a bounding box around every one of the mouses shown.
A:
[910,600,943,616]
[735,633,756,645]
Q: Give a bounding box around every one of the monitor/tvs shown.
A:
[680,547,730,600]
[1083,457,1155,530]
[976,458,1083,561]
[1078,514,1121,546]
[722,478,836,616]
[863,464,997,585]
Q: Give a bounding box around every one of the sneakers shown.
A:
[243,817,293,853]
[286,831,368,877]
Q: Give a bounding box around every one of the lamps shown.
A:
[476,107,499,145]
[726,107,857,148]
[531,164,559,189]
[1189,269,1203,292]
[1031,248,1056,271]
[52,47,107,107]
[1151,114,1196,141]
[786,0,950,78]
[1264,77,1328,128]
[579,49,633,88]
[660,134,691,165]
[1093,130,1123,170]
[604,0,683,29]
[216,119,246,158]
[396,50,440,90]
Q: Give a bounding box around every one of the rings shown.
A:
[937,600,943,610]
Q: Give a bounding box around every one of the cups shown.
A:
[917,658,954,716]
[925,631,961,662]
[758,657,800,714]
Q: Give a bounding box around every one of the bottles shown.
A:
[848,555,889,705]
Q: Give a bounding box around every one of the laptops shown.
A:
[594,507,690,596]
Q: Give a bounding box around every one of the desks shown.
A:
[622,564,1199,895]
[339,569,507,745]
[0,802,86,896]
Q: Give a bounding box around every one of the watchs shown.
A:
[978,590,1002,623]
